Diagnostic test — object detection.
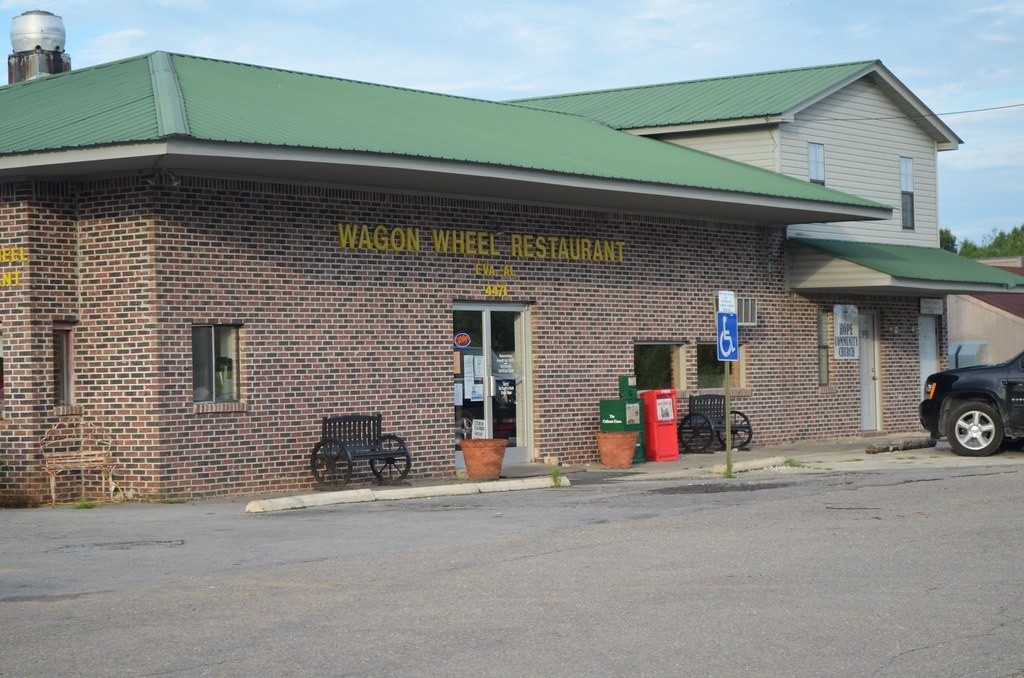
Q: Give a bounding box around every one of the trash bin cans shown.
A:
[640,388,680,461]
[599,398,648,465]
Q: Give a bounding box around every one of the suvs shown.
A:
[919,349,1024,456]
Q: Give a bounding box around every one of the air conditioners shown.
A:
[714,297,758,327]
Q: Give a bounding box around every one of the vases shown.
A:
[459,438,509,480]
[595,431,640,470]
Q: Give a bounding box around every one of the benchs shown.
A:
[42,450,121,507]
[311,414,412,489]
[678,394,753,455]
